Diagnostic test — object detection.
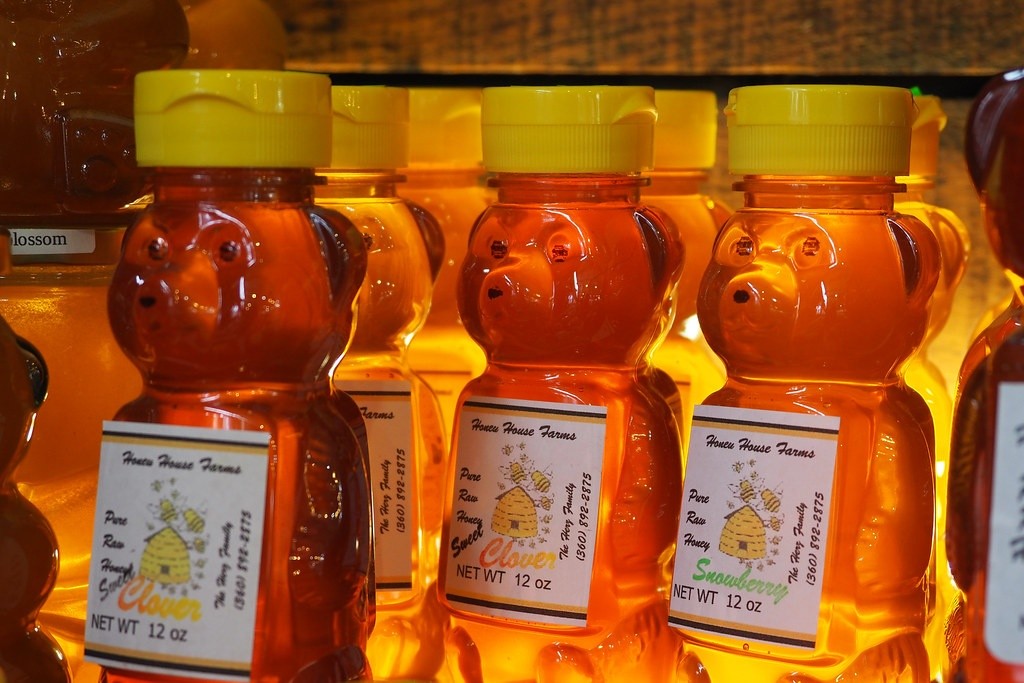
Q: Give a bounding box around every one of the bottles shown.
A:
[0,0,1024,683]
